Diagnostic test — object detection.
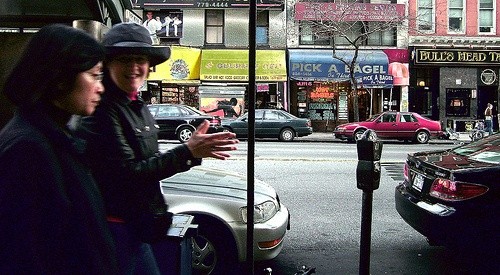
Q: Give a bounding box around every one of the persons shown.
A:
[143,11,182,37]
[276,102,285,111]
[203,98,242,118]
[485,104,493,135]
[79,22,239,275]
[180,98,184,104]
[0,24,121,275]
[254,98,262,109]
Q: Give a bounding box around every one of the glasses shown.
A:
[87,71,104,83]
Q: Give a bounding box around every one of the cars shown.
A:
[222,108,314,142]
[159,165,291,275]
[394,131,498,273]
[333,112,442,145]
[145,104,223,143]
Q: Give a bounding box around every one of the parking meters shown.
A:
[353,129,383,275]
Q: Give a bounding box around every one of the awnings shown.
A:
[288,49,389,84]
[161,79,200,85]
[200,49,287,81]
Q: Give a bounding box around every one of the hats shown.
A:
[102,23,171,67]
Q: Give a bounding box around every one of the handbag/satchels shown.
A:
[126,210,174,244]
